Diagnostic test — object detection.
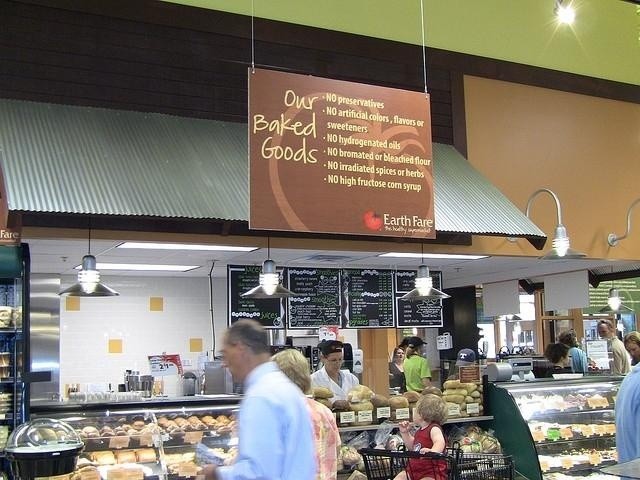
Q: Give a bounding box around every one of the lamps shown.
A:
[239,235,301,300]
[59,239,121,297]
[598,287,635,313]
[506,188,571,260]
[400,253,451,300]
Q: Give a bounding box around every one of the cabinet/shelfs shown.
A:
[338,414,506,479]
[488,375,626,480]
[30,392,246,479]
[0,240,31,467]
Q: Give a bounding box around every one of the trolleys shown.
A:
[356,447,515,480]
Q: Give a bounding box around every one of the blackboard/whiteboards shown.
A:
[226,263,445,330]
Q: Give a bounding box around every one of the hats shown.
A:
[408,336,428,348]
[455,348,476,366]
[317,340,344,354]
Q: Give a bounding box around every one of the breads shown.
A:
[313,378,484,427]
[33,413,239,480]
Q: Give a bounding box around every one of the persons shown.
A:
[599,319,631,376]
[273,347,341,480]
[389,335,432,393]
[558,328,588,375]
[613,361,639,480]
[196,317,318,479]
[623,330,640,364]
[392,392,448,480]
[311,340,361,400]
[543,341,573,376]
[446,348,476,380]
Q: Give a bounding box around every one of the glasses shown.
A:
[325,357,345,364]
